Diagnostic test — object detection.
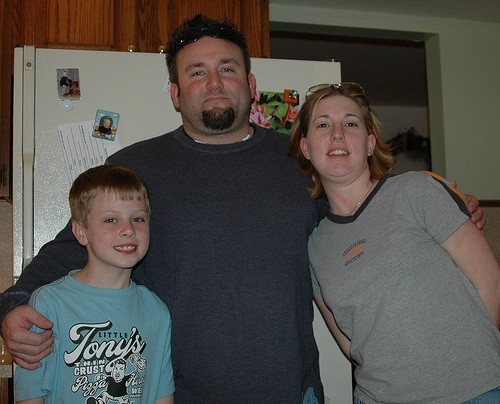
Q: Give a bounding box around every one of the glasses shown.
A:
[305,82,365,103]
[171,25,239,55]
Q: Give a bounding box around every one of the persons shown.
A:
[290,81,500,404]
[12,166,176,404]
[94,115,117,139]
[60,71,71,96]
[0,14,487,404]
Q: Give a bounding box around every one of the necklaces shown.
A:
[333,181,374,218]
[193,127,253,145]
